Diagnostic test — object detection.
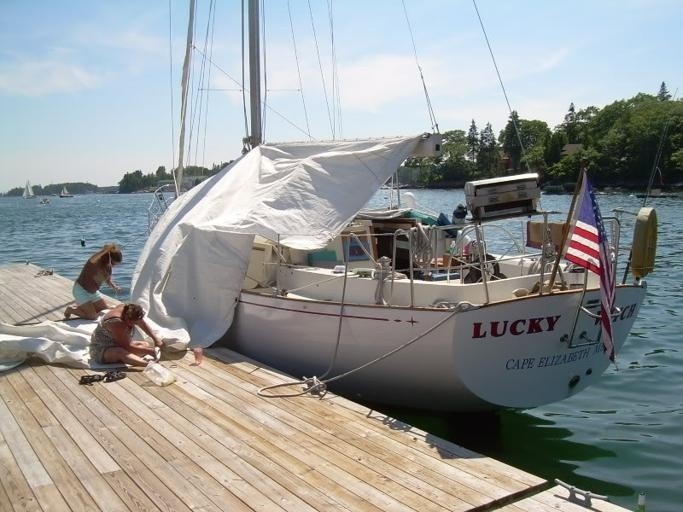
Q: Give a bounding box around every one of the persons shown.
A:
[64,244,122,320]
[90,303,163,368]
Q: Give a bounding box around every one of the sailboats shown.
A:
[59,186,73,198]
[22,182,33,199]
[160,1,657,409]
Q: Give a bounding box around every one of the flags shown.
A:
[561,171,616,363]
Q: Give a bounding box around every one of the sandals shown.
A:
[103,370,127,382]
[78,375,104,385]
